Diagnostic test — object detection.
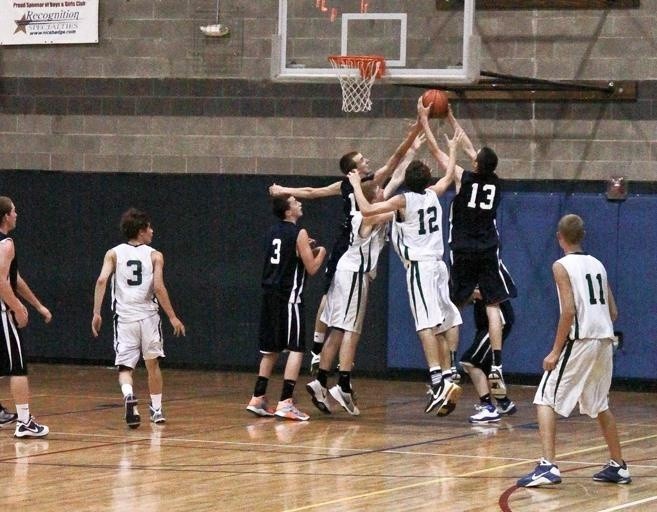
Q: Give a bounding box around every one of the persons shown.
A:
[245,194,328,422]
[0,195,50,438]
[269,96,520,422]
[91,207,186,430]
[0,271,55,426]
[518,214,631,488]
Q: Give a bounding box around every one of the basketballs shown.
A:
[422,90,448,119]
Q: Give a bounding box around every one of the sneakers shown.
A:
[592,459,632,484]
[13,418,49,438]
[488,363,507,400]
[494,399,518,415]
[450,371,461,383]
[327,383,361,416]
[274,397,310,420]
[310,354,320,377]
[305,378,332,415]
[436,382,464,416]
[148,402,165,424]
[123,393,141,429]
[516,456,561,489]
[469,402,502,423]
[245,394,276,418]
[0,407,18,427]
[423,377,453,414]
